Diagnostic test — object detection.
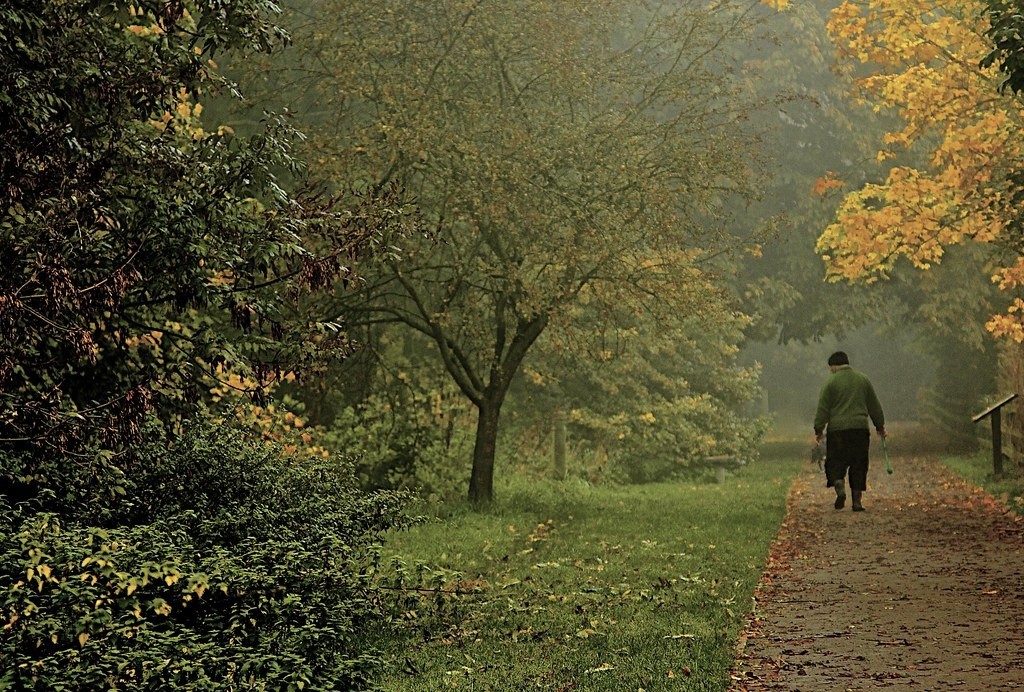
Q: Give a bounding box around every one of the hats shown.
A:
[828,351,849,365]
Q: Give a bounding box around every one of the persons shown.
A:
[814,352,888,512]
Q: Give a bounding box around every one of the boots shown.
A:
[852,489,865,511]
[835,479,846,509]
